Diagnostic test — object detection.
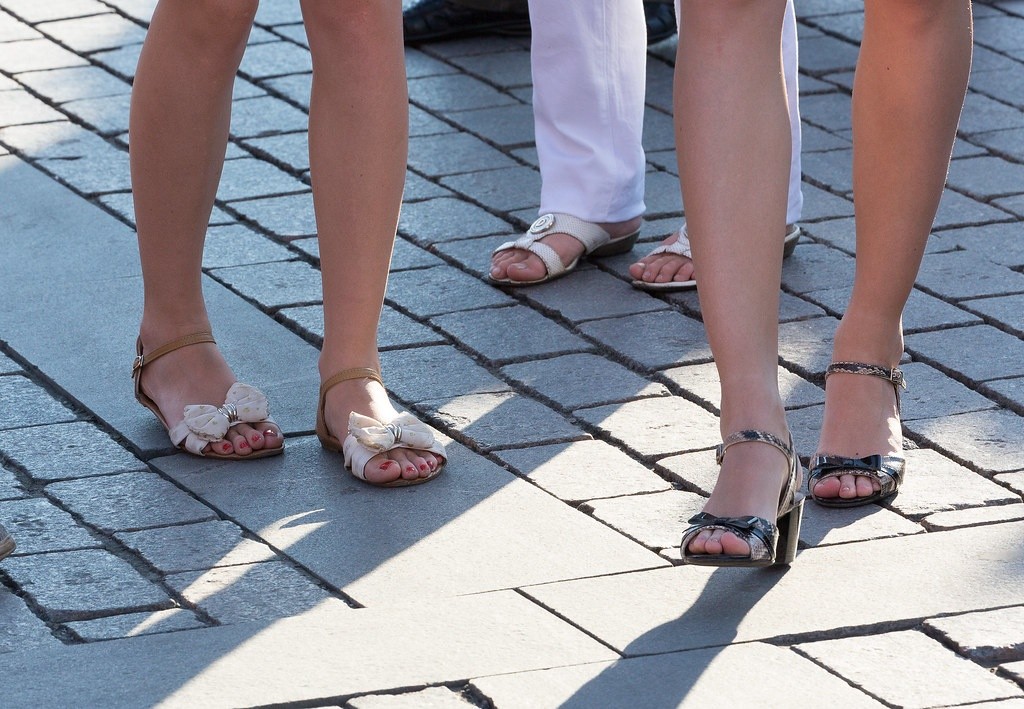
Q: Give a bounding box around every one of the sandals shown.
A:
[131,333,285,460]
[315,368,448,488]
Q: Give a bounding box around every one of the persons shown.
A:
[128,0,447,489]
[486,0,802,290]
[672,0,974,568]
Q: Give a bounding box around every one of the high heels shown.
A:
[680,430,806,568]
[807,361,906,508]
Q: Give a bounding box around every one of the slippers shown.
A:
[488,214,641,288]
[632,223,801,293]
